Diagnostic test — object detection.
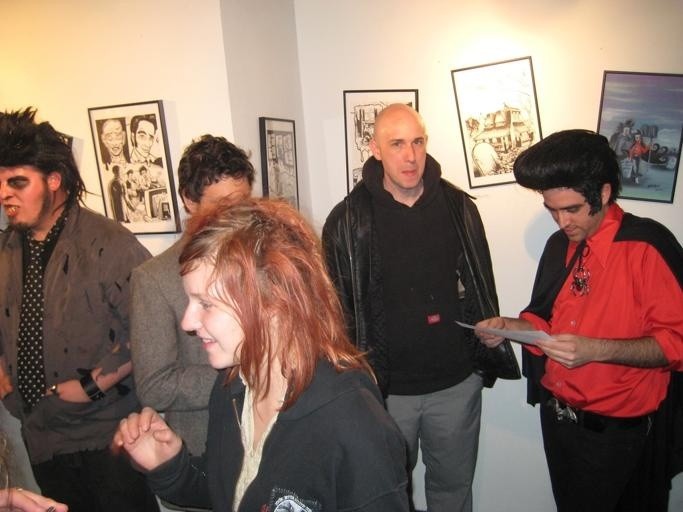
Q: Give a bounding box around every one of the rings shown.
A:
[49,384,57,394]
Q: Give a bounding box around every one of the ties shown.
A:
[15,240,46,409]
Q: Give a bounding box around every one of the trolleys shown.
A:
[619,158,641,186]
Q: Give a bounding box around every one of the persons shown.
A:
[321,103,521,512]
[474,129,683,512]
[128,135,254,512]
[101,113,171,223]
[628,133,650,185]
[0,488,69,512]
[114,188,410,512]
[0,106,161,512]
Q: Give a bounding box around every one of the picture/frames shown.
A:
[344,89,418,195]
[597,70,682,203]
[451,56,543,188]
[259,117,299,211]
[88,100,180,233]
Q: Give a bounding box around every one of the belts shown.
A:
[538,389,641,428]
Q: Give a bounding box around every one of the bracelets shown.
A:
[80,374,106,400]
